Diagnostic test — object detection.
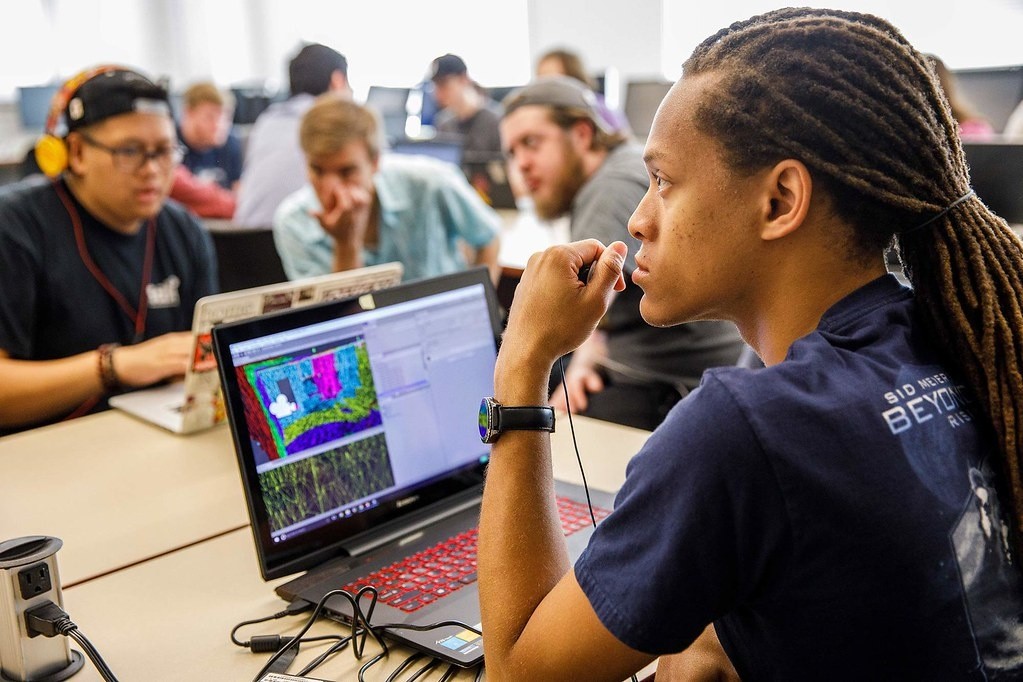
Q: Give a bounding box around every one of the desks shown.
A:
[496,196,570,277]
[0,404,658,682]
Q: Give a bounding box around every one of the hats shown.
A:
[501,75,615,136]
[415,54,466,86]
[59,70,171,139]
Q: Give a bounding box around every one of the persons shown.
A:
[477,7,1023,682]
[172,44,600,230]
[0,65,219,431]
[495,76,761,432]
[922,52,995,138]
[272,101,506,289]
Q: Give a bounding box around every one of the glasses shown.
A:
[81,133,188,172]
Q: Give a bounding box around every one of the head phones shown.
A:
[35,64,155,179]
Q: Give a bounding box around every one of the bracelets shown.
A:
[98,342,121,396]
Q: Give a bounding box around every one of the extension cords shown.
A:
[0,534,85,682]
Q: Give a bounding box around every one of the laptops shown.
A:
[212,265,621,669]
[107,260,406,435]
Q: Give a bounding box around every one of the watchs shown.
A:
[478,397,556,444]
